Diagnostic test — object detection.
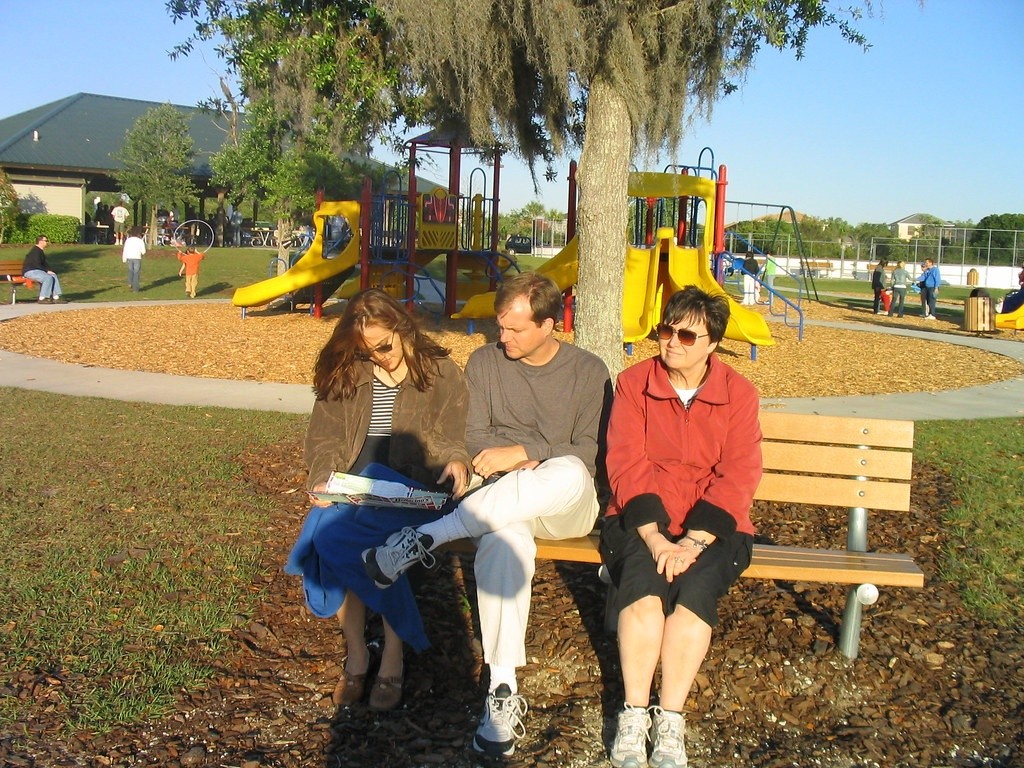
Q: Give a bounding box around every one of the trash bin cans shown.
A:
[964,288,995,332]
[967,268,979,285]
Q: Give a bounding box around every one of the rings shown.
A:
[481,467,487,472]
[676,557,682,562]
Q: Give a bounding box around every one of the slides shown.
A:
[450,232,577,319]
[336,251,439,299]
[233,236,359,306]
[666,243,775,346]
[621,242,660,343]
[996,304,1024,330]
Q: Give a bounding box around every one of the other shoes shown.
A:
[115,241,123,246]
[371,654,405,712]
[332,651,372,706]
[51,297,67,304]
[925,312,936,320]
[38,298,53,305]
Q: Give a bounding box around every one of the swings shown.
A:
[735,205,768,268]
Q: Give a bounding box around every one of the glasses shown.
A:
[40,240,48,243]
[358,329,396,362]
[655,320,710,347]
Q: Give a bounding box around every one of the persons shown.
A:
[872,257,891,315]
[741,251,760,305]
[112,201,130,245]
[912,258,940,319]
[176,245,205,299]
[362,274,613,753]
[122,226,146,292]
[94,202,114,243]
[889,261,916,317]
[602,286,763,761]
[22,235,68,304]
[283,287,469,707]
[764,250,776,303]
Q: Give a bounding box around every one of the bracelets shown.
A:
[685,534,708,551]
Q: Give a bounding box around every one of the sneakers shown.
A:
[361,523,436,589]
[649,702,686,768]
[609,702,651,768]
[474,692,529,757]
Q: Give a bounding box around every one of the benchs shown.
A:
[0,260,37,305]
[332,412,924,660]
